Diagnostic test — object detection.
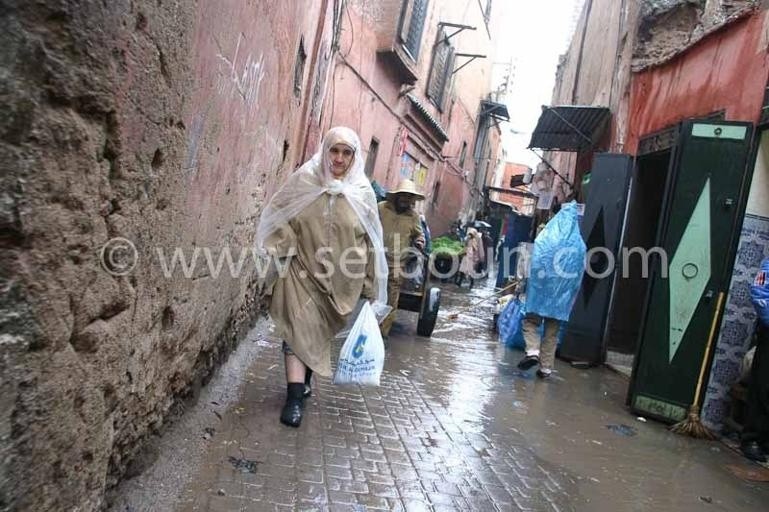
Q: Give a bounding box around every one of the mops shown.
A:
[666,292,728,442]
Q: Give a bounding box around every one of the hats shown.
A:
[386,179,425,203]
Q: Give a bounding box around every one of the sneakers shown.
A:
[517,355,539,371]
[536,368,550,380]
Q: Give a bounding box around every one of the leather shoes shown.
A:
[280,406,302,427]
[302,383,312,398]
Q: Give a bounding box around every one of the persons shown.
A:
[516,201,587,378]
[737,255,769,463]
[438,218,505,290]
[377,178,427,351]
[250,127,388,425]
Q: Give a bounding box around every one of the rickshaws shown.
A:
[397,243,442,336]
